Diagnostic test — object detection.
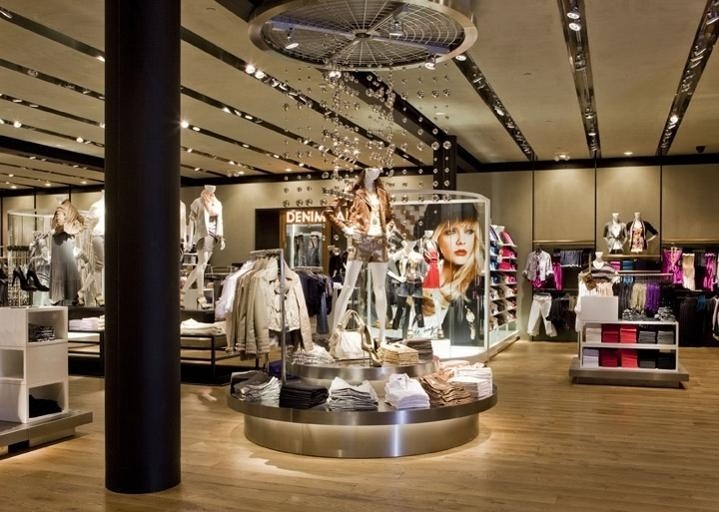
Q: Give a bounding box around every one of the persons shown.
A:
[74,194,107,309]
[627,212,658,253]
[48,194,85,308]
[424,202,482,347]
[581,250,621,285]
[324,168,395,351]
[183,184,225,306]
[603,212,628,255]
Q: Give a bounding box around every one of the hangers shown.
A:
[250,248,281,263]
[536,244,541,253]
[559,292,571,301]
[534,288,550,297]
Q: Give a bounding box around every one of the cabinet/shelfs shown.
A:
[0,305,70,423]
[489,240,519,334]
[580,320,679,372]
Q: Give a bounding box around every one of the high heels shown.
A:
[12,263,49,291]
